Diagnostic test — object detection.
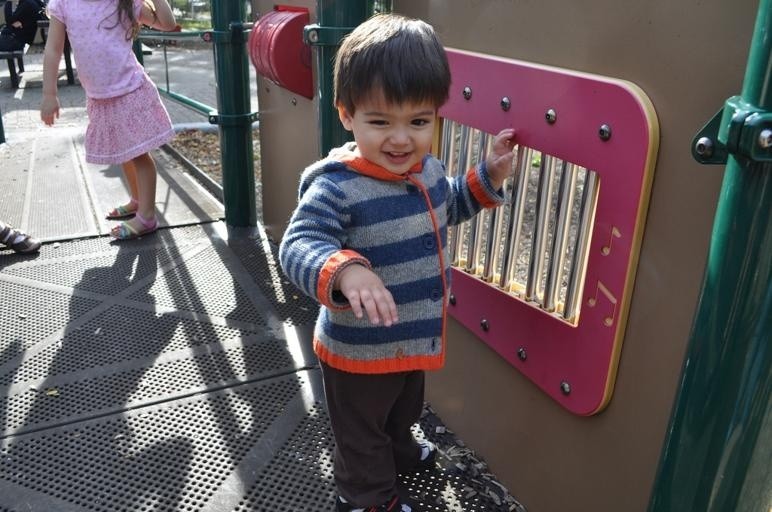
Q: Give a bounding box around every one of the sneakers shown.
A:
[1,226,41,254]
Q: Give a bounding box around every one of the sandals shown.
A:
[417,441,437,463]
[107,201,137,219]
[110,213,159,240]
[336,493,412,512]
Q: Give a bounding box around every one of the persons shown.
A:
[3,0,43,51]
[40,0,177,240]
[279,14,515,512]
[0,221,42,255]
